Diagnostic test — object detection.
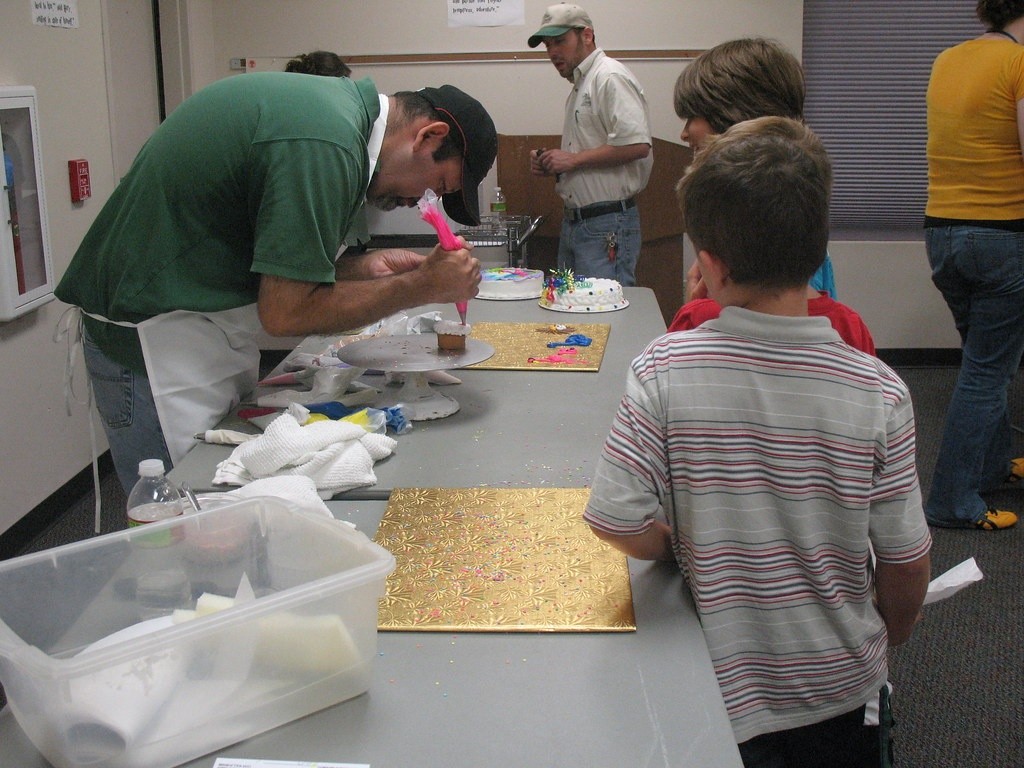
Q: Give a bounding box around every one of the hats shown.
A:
[528,2,593,48]
[415,84,498,227]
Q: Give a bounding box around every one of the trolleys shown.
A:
[453,214,543,269]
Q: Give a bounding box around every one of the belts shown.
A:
[564,198,635,224]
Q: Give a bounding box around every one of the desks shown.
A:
[0,287,746,768]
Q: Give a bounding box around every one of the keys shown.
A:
[606,231,618,256]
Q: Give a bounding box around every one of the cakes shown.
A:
[478,267,544,298]
[542,262,624,311]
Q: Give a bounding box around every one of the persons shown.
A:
[53,50,499,502]
[582,37,933,768]
[527,4,654,288]
[923,0,1024,530]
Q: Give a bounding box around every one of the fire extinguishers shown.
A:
[5,157,27,299]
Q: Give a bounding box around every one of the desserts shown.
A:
[433,320,471,350]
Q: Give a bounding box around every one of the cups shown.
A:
[182,493,265,598]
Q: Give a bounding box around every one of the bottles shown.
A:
[127,458,192,621]
[490,187,507,236]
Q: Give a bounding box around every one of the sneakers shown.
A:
[967,505,1017,529]
[1007,458,1024,482]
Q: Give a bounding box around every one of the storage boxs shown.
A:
[0,496,395,768]
